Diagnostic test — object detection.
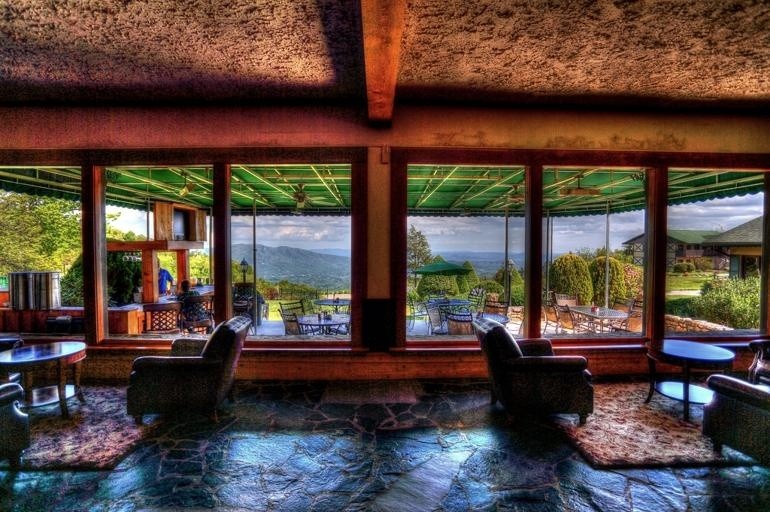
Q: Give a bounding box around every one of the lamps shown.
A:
[178,172,197,198]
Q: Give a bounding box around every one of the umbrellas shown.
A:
[412,262,475,277]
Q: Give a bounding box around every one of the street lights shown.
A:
[239,257,249,282]
[506,256,514,306]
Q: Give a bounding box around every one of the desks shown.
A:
[643,339,736,420]
[0,342,88,419]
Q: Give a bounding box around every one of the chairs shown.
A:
[0,383,32,478]
[701,374,770,470]
[745,339,769,383]
[277,297,352,337]
[422,289,638,337]
[0,338,27,386]
[145,281,260,336]
[122,316,255,426]
[473,316,594,437]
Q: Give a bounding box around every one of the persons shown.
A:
[158,257,174,296]
[176,279,214,334]
